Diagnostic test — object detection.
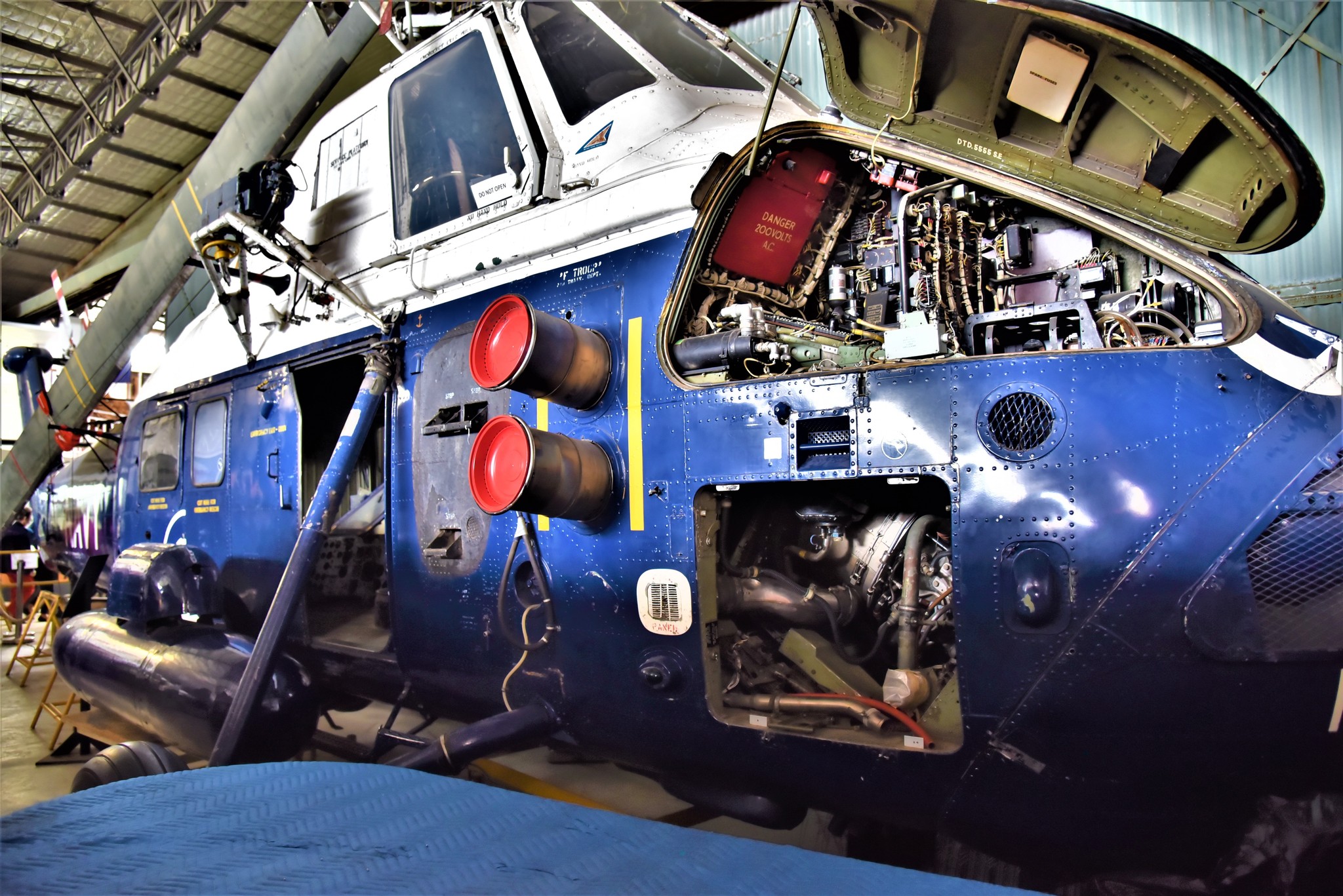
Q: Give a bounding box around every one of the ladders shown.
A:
[4,591,71,687]
[29,670,89,752]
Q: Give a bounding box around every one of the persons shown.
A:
[0,507,54,621]
